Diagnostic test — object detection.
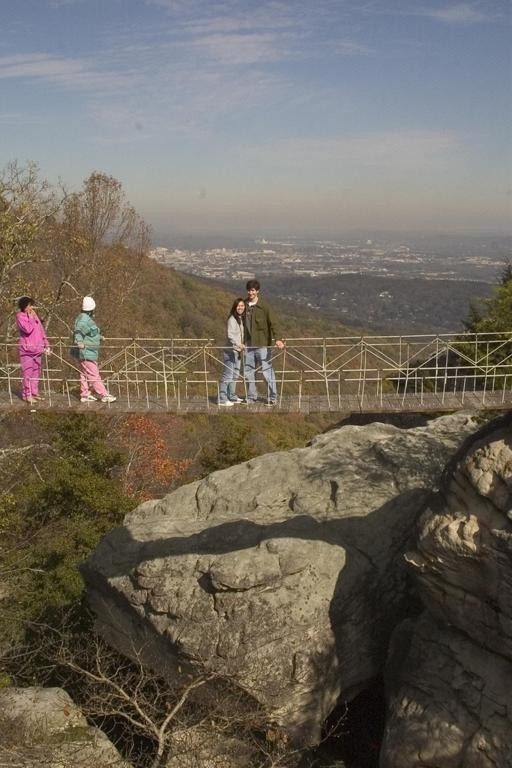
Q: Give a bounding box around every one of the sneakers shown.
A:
[263,399,278,408]
[79,395,99,403]
[240,398,259,406]
[230,397,246,404]
[216,401,235,407]
[22,395,46,404]
[101,394,117,403]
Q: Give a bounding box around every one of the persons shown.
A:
[72,295,117,403]
[240,280,284,407]
[15,296,50,405]
[217,297,246,407]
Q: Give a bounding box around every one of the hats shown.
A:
[82,295,96,312]
